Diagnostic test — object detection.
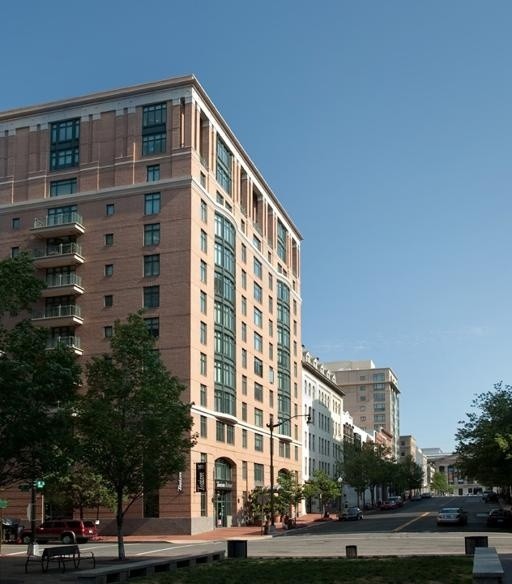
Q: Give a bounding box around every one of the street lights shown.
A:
[265,412,312,534]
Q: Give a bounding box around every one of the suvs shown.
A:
[19,519,98,544]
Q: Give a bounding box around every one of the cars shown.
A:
[436,507,468,527]
[379,496,404,510]
[337,506,364,520]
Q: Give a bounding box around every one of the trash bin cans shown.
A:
[465,536,488,554]
[228,540,247,559]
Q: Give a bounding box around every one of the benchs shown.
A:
[24,545,96,574]
[472,546,504,582]
[71,547,226,583]
